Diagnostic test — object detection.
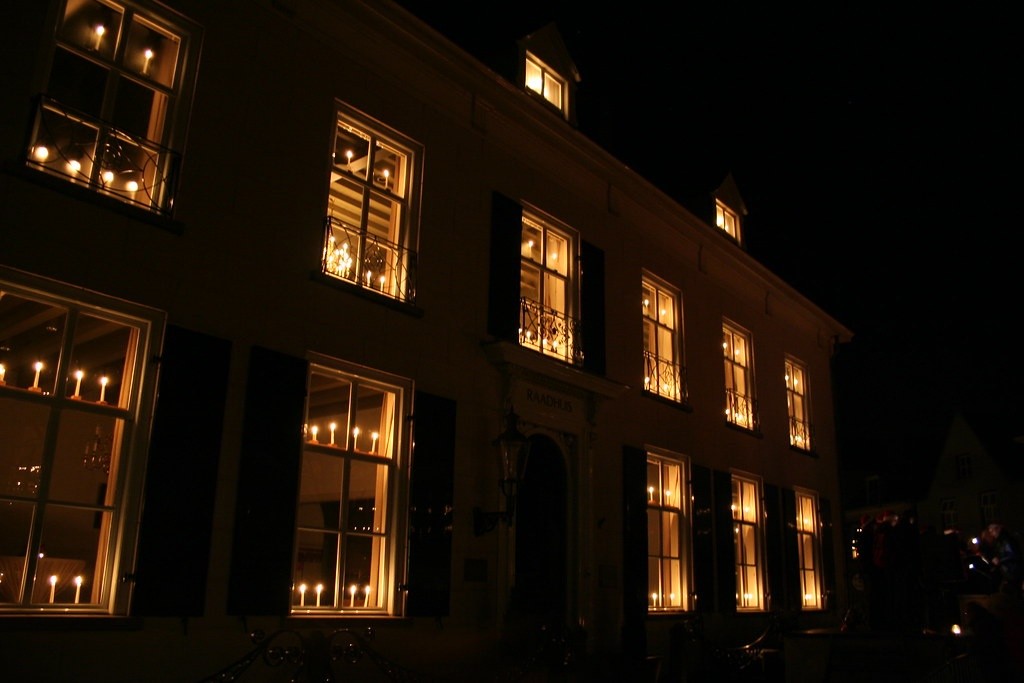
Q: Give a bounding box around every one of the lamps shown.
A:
[82,423,113,476]
[473,404,532,537]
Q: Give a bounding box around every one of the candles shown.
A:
[328,422,337,444]
[651,593,658,607]
[99,376,110,402]
[325,251,397,296]
[299,583,308,606]
[33,361,44,388]
[95,26,105,50]
[370,431,379,453]
[349,584,358,607]
[143,50,153,73]
[364,585,371,607]
[49,575,59,603]
[644,299,649,314]
[353,426,360,450]
[315,583,324,606]
[666,491,670,506]
[344,150,353,170]
[310,424,319,442]
[74,575,84,604]
[74,370,85,396]
[648,486,655,501]
[382,170,389,188]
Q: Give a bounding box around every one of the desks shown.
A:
[0,556,94,603]
